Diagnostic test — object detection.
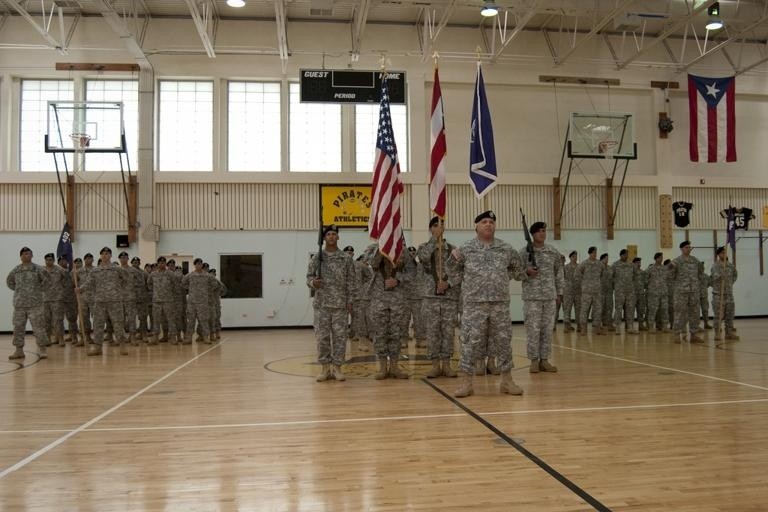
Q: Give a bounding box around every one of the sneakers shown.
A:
[675,320,740,343]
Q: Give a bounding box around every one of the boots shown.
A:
[616,322,673,334]
[528,359,556,372]
[9,347,25,359]
[104,329,221,346]
[389,358,408,379]
[38,344,47,358]
[475,356,500,375]
[563,323,614,336]
[87,343,102,355]
[442,358,457,377]
[376,357,388,379]
[332,364,345,381]
[455,376,473,397]
[120,342,128,355]
[358,336,369,351]
[47,336,93,346]
[500,371,523,394]
[427,358,440,378]
[316,363,331,381]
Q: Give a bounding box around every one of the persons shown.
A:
[341,244,502,377]
[417,213,463,380]
[513,220,569,377]
[363,239,419,381]
[442,207,524,400]
[306,224,358,381]
[556,240,741,344]
[6,240,229,362]
[684,70,740,166]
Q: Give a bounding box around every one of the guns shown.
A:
[520,207,538,270]
[318,206,323,277]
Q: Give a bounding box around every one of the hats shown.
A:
[100,247,111,254]
[600,254,608,260]
[475,211,496,223]
[654,253,662,259]
[45,253,93,263]
[530,222,546,233]
[323,224,338,237]
[633,258,641,262]
[20,247,30,256]
[343,246,354,252]
[146,257,183,271]
[588,247,595,253]
[119,252,128,258]
[429,216,444,228]
[680,241,690,248]
[620,249,626,255]
[717,246,724,254]
[569,251,576,257]
[131,257,140,263]
[194,258,216,273]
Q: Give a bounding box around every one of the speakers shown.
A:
[117,234,129,248]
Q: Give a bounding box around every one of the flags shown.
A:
[467,58,502,201]
[53,218,75,269]
[367,69,407,271]
[427,64,450,219]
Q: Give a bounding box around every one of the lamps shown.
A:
[480,0,498,18]
[704,2,724,30]
